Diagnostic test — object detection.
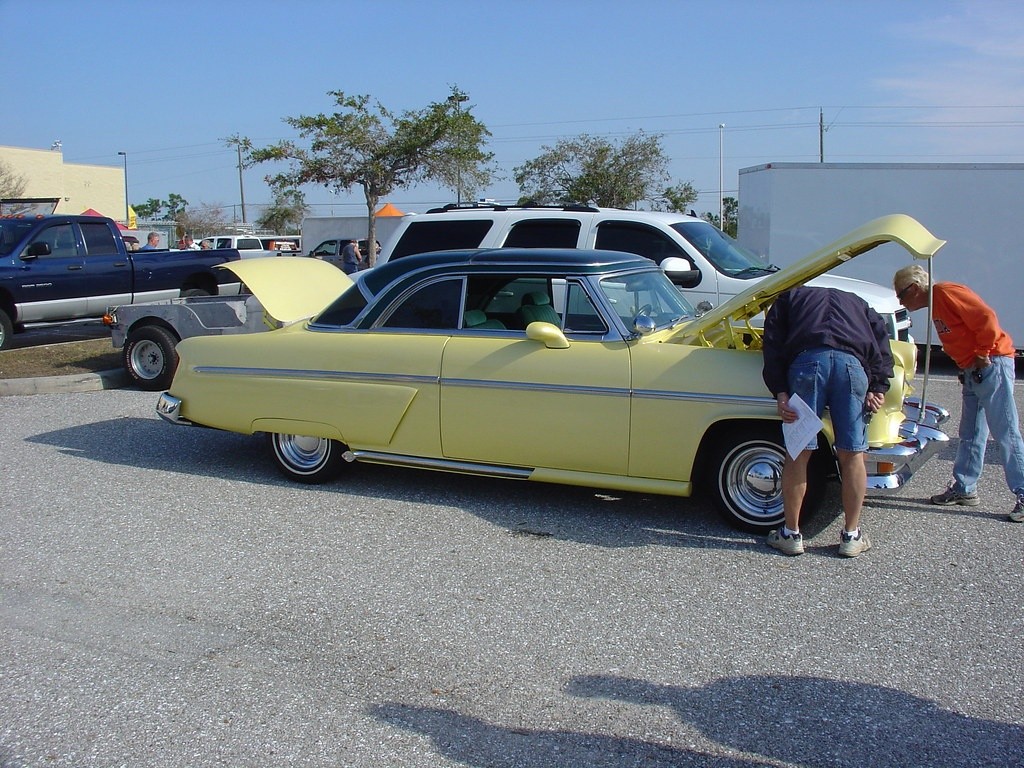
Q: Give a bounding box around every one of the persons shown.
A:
[342,240,362,273]
[894,266,1024,521]
[180,236,211,250]
[763,285,895,559]
[140,232,159,249]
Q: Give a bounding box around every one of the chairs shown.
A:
[463,310,507,331]
[52,231,78,255]
[515,292,562,332]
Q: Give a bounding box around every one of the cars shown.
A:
[155,214,951,538]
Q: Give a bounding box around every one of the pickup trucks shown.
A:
[0,214,242,348]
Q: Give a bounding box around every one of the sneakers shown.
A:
[838,528,871,557]
[767,527,804,555]
[931,487,980,506]
[1009,501,1024,522]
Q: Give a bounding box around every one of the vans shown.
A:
[193,235,264,254]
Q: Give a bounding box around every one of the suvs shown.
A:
[371,201,918,367]
[303,238,383,276]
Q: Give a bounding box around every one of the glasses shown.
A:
[896,281,918,300]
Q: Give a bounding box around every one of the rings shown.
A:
[783,410,785,413]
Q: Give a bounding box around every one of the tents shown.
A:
[374,203,404,216]
[79,208,127,230]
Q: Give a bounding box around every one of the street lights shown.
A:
[718,123,726,235]
[118,151,130,223]
